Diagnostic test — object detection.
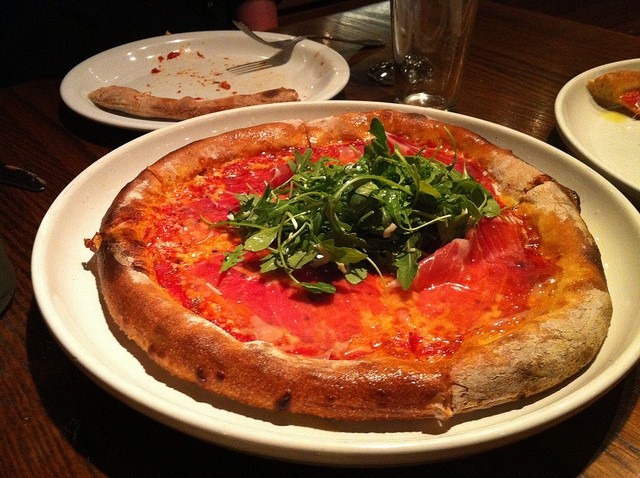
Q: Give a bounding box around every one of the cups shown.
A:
[391,0,474,108]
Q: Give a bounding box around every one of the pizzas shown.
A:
[589,69,640,119]
[87,85,300,121]
[85,110,612,423]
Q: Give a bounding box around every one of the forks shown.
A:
[233,19,385,50]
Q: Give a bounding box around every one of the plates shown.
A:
[59,31,350,132]
[30,100,640,466]
[554,58,640,193]
